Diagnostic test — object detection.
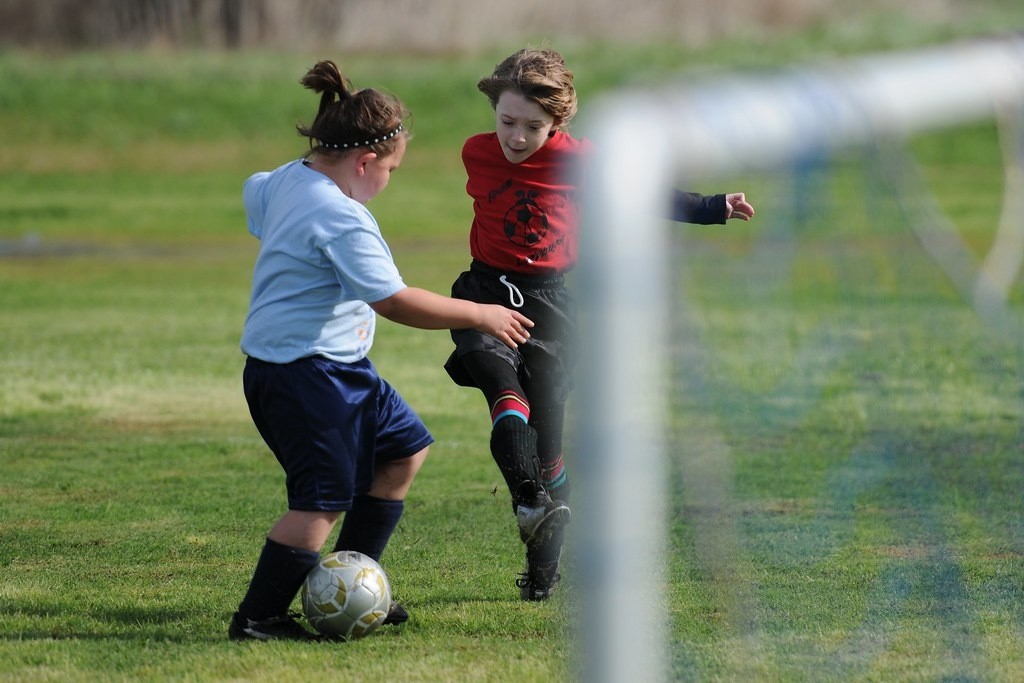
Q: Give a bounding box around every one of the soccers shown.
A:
[300,550,392,642]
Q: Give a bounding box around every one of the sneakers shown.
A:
[511,453,573,557]
[516,559,561,600]
[227,614,319,644]
[382,602,408,627]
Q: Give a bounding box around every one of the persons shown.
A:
[228,60,535,642]
[449,49,755,601]
